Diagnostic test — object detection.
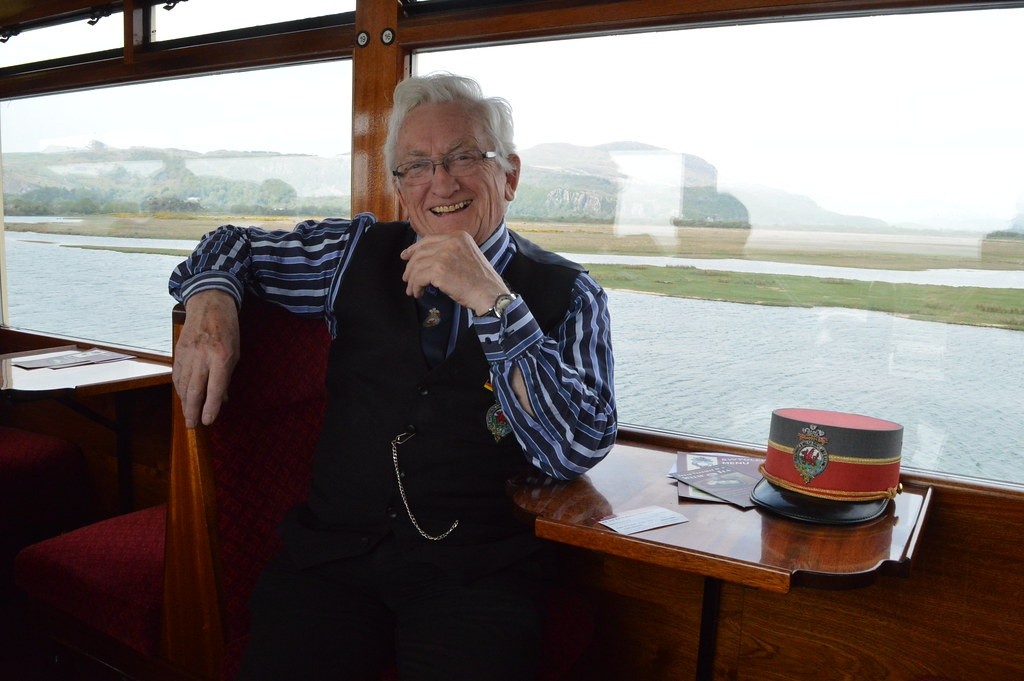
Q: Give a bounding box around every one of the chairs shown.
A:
[180,292,334,681]
[12,301,182,681]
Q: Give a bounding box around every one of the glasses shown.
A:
[392,150,503,186]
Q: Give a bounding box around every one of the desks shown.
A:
[0,343,173,518]
[512,436,936,681]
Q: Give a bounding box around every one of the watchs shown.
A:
[481,293,519,319]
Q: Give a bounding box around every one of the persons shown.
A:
[170,78,616,681]
